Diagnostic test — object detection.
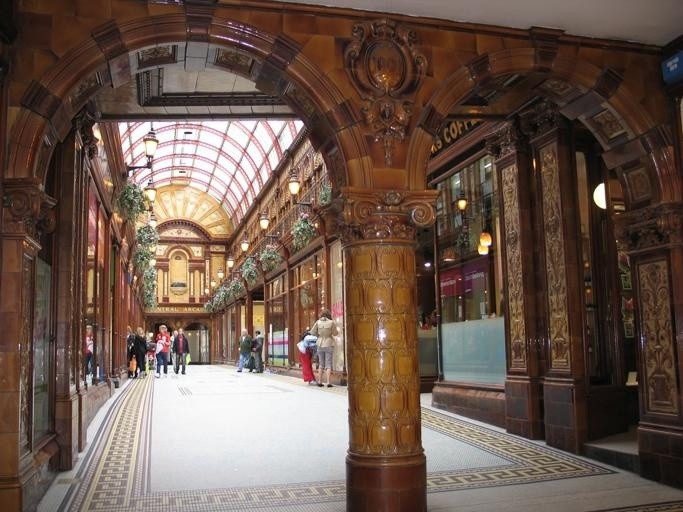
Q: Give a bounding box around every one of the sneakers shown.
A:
[154,373,159,378]
[309,381,317,386]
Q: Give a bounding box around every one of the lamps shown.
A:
[258,211,278,238]
[125,126,159,212]
[224,231,249,273]
[287,168,312,206]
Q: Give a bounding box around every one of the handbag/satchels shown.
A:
[129,357,137,372]
[185,353,190,365]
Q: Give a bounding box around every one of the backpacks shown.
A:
[301,330,315,343]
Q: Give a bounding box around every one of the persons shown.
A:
[126,325,139,380]
[422,315,429,330]
[134,327,148,380]
[172,328,190,375]
[251,330,265,373]
[146,332,158,371]
[309,307,339,387]
[236,329,253,373]
[171,330,180,375]
[296,329,320,387]
[430,310,437,327]
[86,325,96,376]
[154,324,171,379]
